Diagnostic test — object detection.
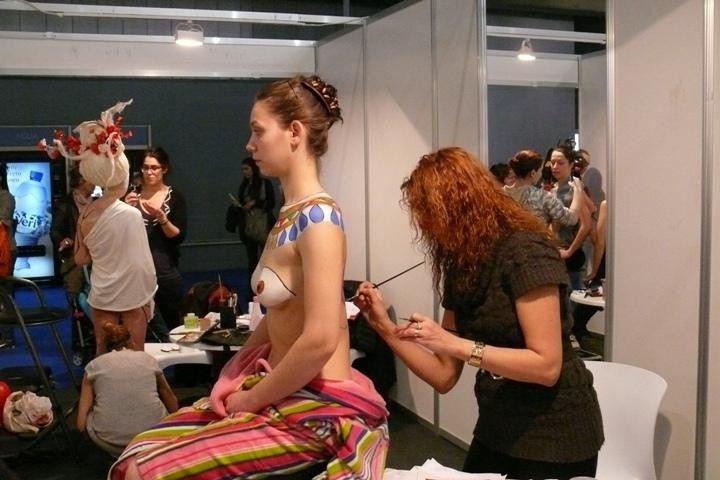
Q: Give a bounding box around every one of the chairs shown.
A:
[568,357,670,480]
[1,275,86,458]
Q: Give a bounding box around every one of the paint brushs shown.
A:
[346,261,427,302]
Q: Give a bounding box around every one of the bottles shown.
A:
[184,313,198,328]
[11,170,48,246]
[250,297,264,331]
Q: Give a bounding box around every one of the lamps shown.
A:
[515,37,539,63]
[173,18,207,50]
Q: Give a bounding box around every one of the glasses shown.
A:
[140,166,160,175]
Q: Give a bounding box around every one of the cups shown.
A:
[220,306,237,327]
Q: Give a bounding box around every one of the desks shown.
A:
[568,283,607,314]
[165,280,360,373]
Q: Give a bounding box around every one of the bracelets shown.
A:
[467,340,488,368]
[158,218,172,226]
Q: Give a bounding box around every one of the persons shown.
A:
[75,323,178,457]
[0,164,21,352]
[229,157,276,302]
[48,166,95,353]
[122,143,187,323]
[352,146,604,478]
[110,75,391,479]
[72,151,154,358]
[488,138,606,343]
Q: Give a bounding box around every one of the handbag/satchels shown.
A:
[243,207,269,241]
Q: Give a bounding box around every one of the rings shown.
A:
[416,320,422,330]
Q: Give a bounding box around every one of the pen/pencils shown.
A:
[166,332,198,335]
